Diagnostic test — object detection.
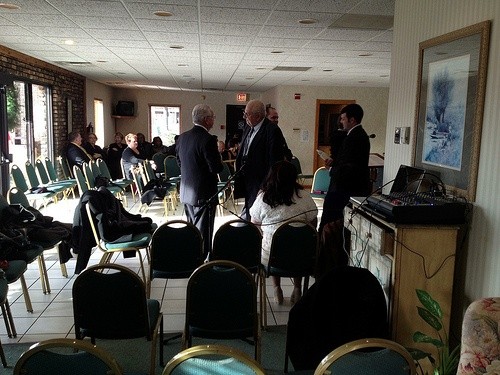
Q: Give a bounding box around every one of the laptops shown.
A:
[390,165,426,193]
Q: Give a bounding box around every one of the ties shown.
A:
[243,128,254,155]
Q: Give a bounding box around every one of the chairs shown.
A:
[0,154,420,375]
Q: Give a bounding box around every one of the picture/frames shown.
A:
[412,21,493,202]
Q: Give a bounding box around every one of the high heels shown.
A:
[290,287,301,306]
[274,286,283,305]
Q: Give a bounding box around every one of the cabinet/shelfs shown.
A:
[343,195,457,375]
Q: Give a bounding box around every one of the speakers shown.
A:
[116,103,134,117]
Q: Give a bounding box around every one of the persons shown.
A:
[68,131,94,178]
[226,139,242,172]
[87,121,94,132]
[265,106,278,125]
[217,140,229,172]
[122,133,157,171]
[107,132,128,179]
[249,162,318,305]
[319,104,370,237]
[83,133,107,167]
[169,135,181,167]
[149,137,168,155]
[234,99,289,221]
[175,104,223,258]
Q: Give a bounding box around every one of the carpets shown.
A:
[0,321,284,375]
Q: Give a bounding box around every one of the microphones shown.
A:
[240,163,247,170]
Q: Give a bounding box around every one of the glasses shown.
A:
[242,112,258,117]
